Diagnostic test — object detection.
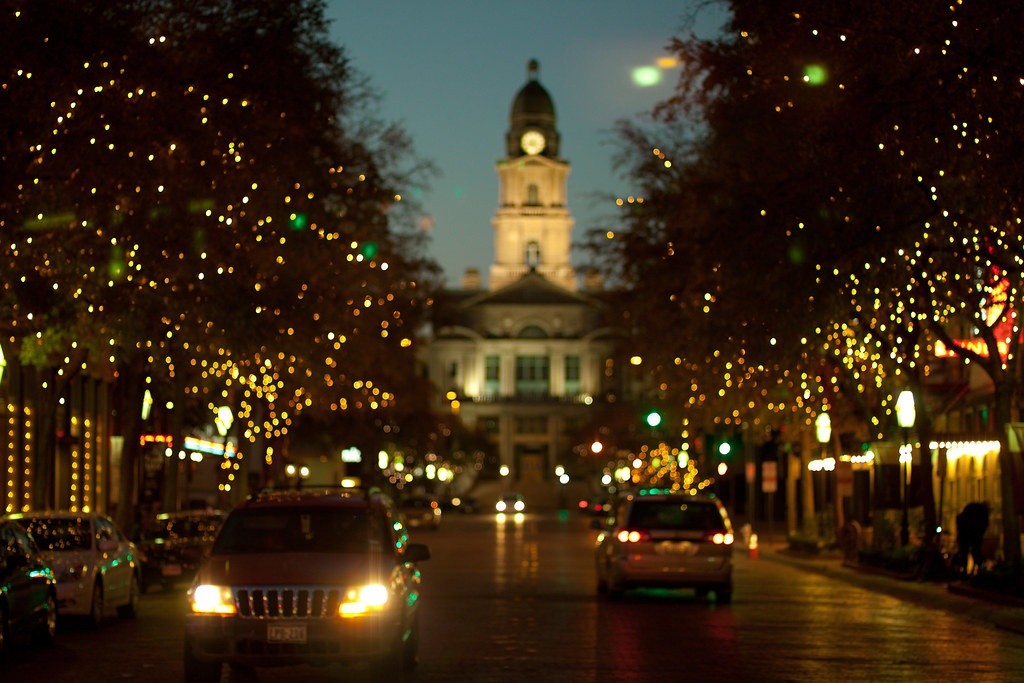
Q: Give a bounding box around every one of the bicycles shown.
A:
[913,526,978,590]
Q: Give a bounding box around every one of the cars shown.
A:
[0,513,143,627]
[443,493,488,515]
[0,518,57,646]
[578,497,609,517]
[181,489,432,683]
[496,492,525,513]
[396,495,442,531]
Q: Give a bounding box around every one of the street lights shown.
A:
[893,386,916,548]
[816,411,831,554]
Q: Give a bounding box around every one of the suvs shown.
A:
[588,494,736,609]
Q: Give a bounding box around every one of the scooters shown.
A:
[133,539,174,593]
